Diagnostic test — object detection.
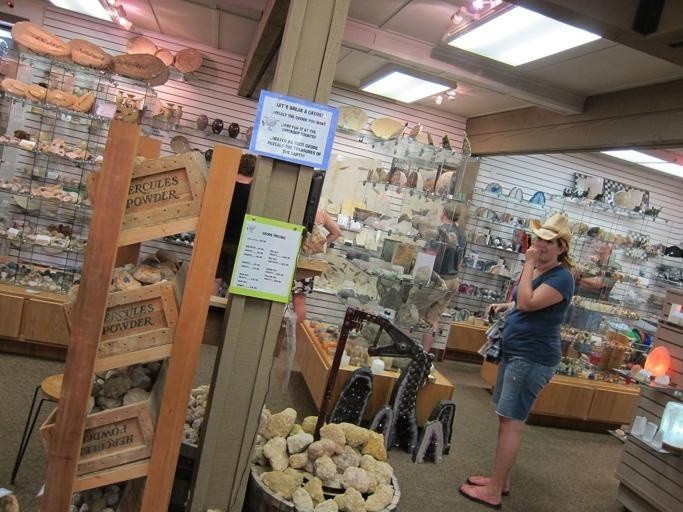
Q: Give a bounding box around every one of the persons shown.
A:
[207,153,257,299]
[560,241,624,359]
[398,204,468,353]
[290,209,344,351]
[456,211,575,509]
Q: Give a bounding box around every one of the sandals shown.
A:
[459,474,509,509]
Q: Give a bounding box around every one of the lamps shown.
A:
[356,63,456,105]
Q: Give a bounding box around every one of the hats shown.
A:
[442,200,462,215]
[530,212,572,242]
